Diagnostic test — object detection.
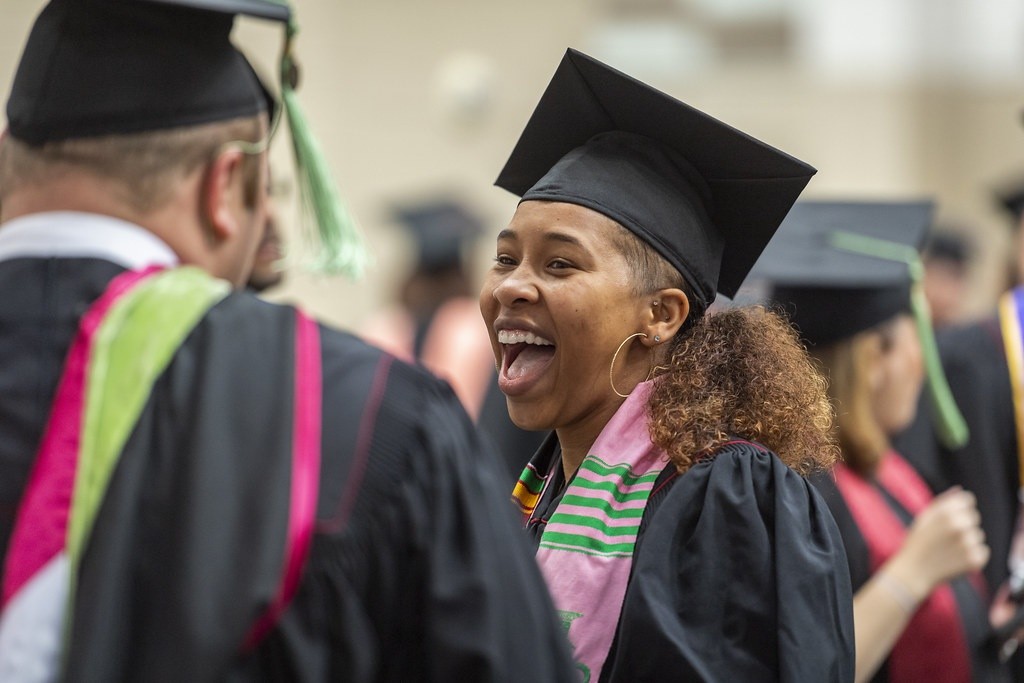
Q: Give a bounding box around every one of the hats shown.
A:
[745,195,968,453]
[493,48,819,316]
[6,0,356,280]
[390,197,483,260]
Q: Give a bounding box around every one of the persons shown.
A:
[0,0,590,681]
[360,194,1024,683]
[479,47,857,681]
